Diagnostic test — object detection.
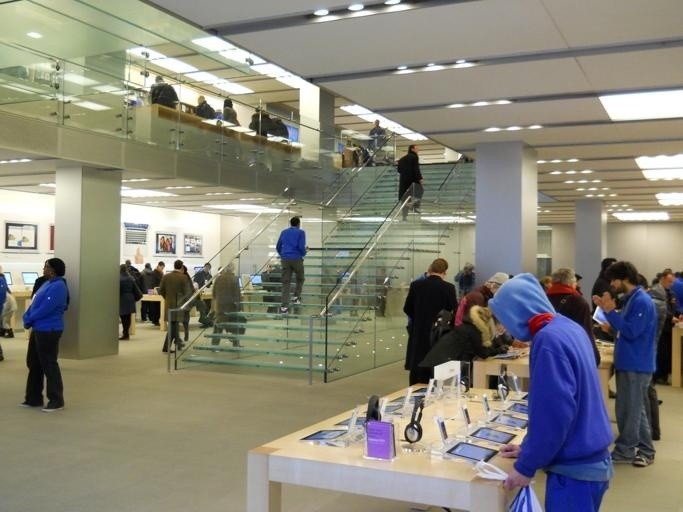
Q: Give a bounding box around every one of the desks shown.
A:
[127,286,262,335]
[133,103,301,172]
[11,291,33,338]
[671,321,683,387]
[384,285,409,315]
[246,383,533,512]
[472,338,613,409]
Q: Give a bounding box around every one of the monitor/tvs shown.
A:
[21,272,39,285]
[249,274,263,286]
[3,272,13,286]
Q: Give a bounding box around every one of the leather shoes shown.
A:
[174,343,185,351]
[161,346,176,354]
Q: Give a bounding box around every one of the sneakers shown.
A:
[609,450,636,465]
[118,334,129,339]
[279,305,289,315]
[40,400,66,413]
[290,296,303,305]
[16,398,44,409]
[632,447,656,467]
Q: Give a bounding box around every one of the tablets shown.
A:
[592,306,618,324]
[298,387,533,464]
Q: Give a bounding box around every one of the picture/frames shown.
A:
[154,231,178,257]
[46,224,54,253]
[182,233,205,258]
[0,217,41,254]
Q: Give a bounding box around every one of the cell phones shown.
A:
[513,375,521,394]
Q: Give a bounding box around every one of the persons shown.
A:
[367,119,387,166]
[149,75,178,109]
[395,143,424,220]
[249,106,271,135]
[215,108,224,119]
[275,216,310,313]
[195,95,215,119]
[271,115,289,138]
[224,99,241,126]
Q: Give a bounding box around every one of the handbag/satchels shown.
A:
[430,308,454,348]
[127,271,143,302]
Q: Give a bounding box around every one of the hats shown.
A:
[486,270,510,286]
